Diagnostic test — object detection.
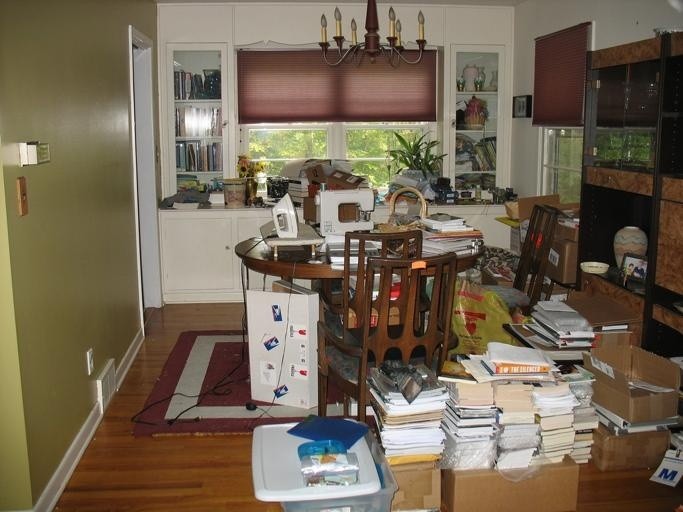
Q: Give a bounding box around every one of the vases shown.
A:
[613,225,648,270]
[246,178,258,202]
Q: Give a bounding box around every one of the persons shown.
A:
[624,262,632,275]
[633,259,646,274]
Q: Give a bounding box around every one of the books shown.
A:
[341,271,398,280]
[348,290,401,299]
[467,135,496,172]
[170,66,225,211]
[327,241,378,251]
[327,249,368,266]
[420,211,485,257]
[342,304,402,330]
[367,295,604,470]
[347,280,401,289]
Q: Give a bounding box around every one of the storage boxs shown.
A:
[494,194,580,284]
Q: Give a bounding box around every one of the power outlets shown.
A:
[86,348,95,376]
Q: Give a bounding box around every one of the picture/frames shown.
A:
[616,252,648,286]
[511,95,531,118]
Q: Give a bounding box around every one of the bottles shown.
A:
[189,70,221,98]
[464,95,486,132]
[457,61,498,91]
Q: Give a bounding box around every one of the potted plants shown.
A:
[384,131,449,191]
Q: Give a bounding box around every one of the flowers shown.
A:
[236,156,267,177]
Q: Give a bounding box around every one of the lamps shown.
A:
[318,1,427,68]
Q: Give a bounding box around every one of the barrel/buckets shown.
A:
[222,182,248,209]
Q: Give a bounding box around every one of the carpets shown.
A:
[129,330,376,438]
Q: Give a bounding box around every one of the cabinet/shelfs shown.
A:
[159,210,233,305]
[579,32,683,336]
[448,44,515,202]
[155,42,234,203]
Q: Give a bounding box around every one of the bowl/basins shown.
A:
[579,261,611,274]
